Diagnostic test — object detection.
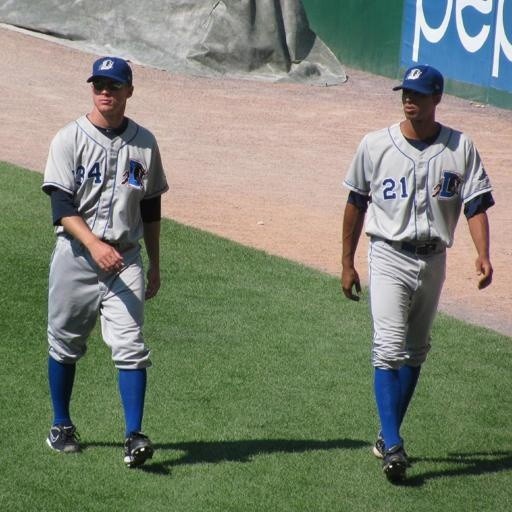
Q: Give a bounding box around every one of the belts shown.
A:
[386,240,435,255]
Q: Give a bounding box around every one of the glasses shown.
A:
[91,79,123,91]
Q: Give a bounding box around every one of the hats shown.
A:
[393,64,443,96]
[87,56,133,86]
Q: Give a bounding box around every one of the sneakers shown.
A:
[46,424,80,452]
[373,430,407,483]
[123,430,154,468]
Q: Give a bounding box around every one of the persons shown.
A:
[42,57,170,467]
[342,66,494,474]
